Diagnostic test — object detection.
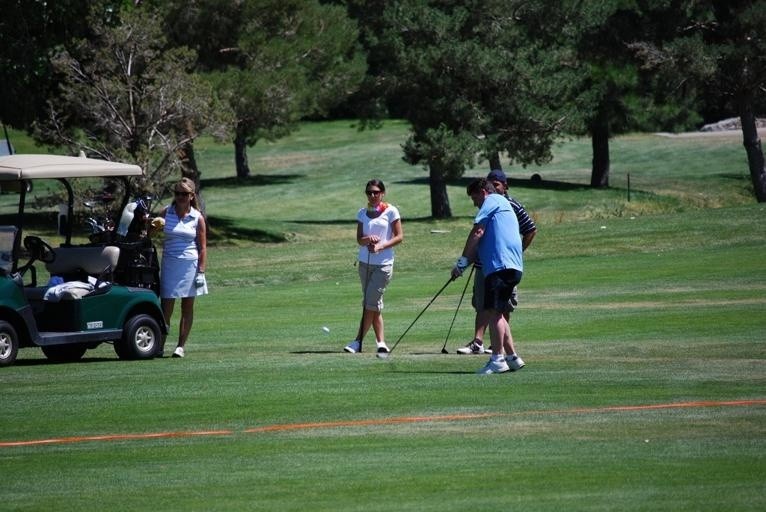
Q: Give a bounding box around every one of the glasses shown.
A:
[173,189,192,197]
[366,190,382,196]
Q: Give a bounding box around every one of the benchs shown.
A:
[25,246,122,300]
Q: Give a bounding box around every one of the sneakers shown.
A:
[457,338,484,354]
[173,346,185,358]
[480,357,510,374]
[343,340,362,353]
[484,345,493,354]
[375,337,390,353]
[504,355,526,370]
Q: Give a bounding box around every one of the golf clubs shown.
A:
[442,261,476,354]
[377,262,473,359]
[82,192,166,240]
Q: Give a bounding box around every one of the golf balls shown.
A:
[322,326,330,333]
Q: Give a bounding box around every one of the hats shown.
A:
[487,170,509,190]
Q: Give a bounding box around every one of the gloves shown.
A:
[196,271,204,288]
[451,267,464,281]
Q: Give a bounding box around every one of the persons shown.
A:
[146,177,207,358]
[457,167,537,353]
[344,179,404,353]
[452,177,527,373]
